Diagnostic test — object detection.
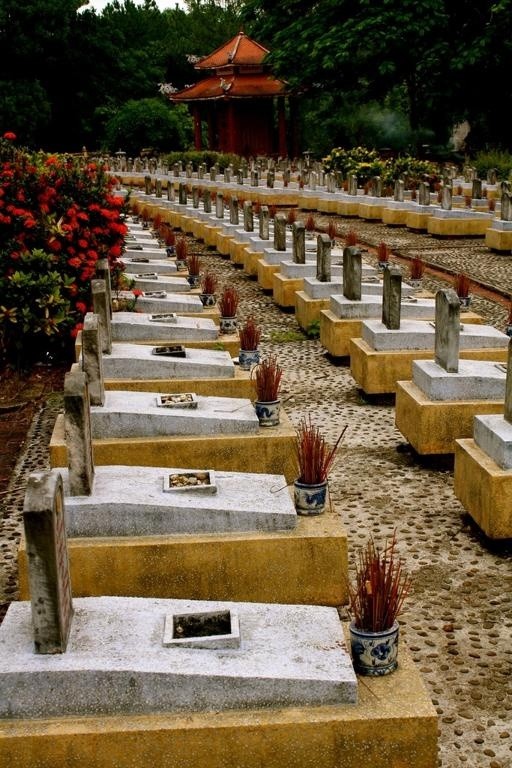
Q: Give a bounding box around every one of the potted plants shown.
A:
[218,286,238,334]
[186,252,202,289]
[142,206,150,229]
[158,223,166,247]
[164,227,177,256]
[152,213,161,237]
[269,203,277,222]
[176,239,188,271]
[327,219,336,249]
[238,319,261,371]
[286,208,297,231]
[305,216,316,240]
[211,191,217,206]
[255,199,261,218]
[286,413,349,516]
[342,527,413,677]
[223,194,230,210]
[506,304,512,336]
[376,240,392,273]
[131,199,141,224]
[405,254,427,293]
[249,352,284,427]
[239,198,246,212]
[200,270,218,309]
[453,272,471,313]
